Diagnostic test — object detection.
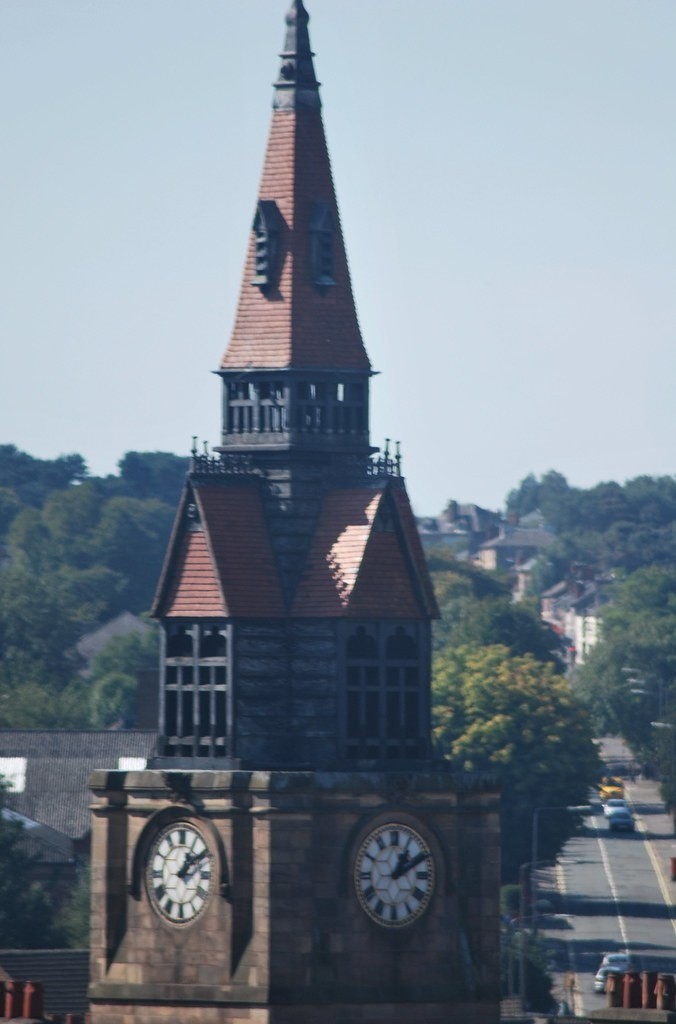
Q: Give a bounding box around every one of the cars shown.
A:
[598,775,626,800]
[593,967,623,994]
[602,798,630,817]
[599,953,630,973]
[608,810,637,832]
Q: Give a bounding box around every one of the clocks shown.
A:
[145,821,215,926]
[353,823,435,928]
